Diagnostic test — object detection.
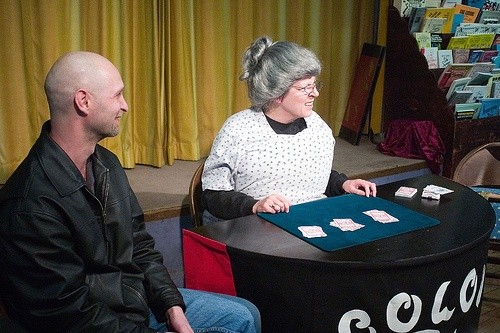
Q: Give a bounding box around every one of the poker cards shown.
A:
[395,186,418,198]
[363,209,399,224]
[297,226,327,238]
[330,218,365,232]
[421,184,454,200]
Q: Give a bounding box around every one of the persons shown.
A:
[201,36,377,225]
[0,52,263,333]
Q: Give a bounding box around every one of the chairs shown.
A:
[451,141,500,286]
[189,164,205,228]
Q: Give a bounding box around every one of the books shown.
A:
[394,0,500,120]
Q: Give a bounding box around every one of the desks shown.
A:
[187,172,497,333]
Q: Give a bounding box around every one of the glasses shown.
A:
[292,80,324,93]
